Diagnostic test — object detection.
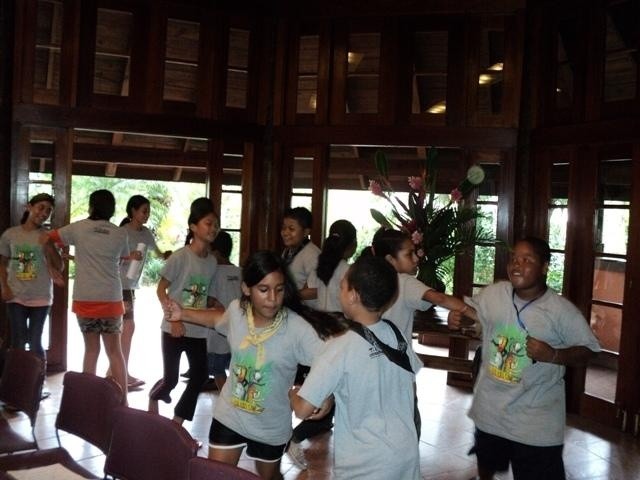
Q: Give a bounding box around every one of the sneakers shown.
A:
[128,379,145,387]
[288,440,307,470]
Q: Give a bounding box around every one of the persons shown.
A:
[106,195,172,388]
[447,237,602,479]
[149,197,480,479]
[0,193,65,413]
[41,189,128,407]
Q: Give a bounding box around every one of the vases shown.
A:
[414,260,446,315]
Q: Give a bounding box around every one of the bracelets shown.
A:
[549,350,559,365]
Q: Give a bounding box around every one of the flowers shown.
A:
[363,144,515,301]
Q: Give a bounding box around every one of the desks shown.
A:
[410,304,483,391]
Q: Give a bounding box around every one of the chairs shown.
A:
[183,455,263,480]
[100,405,198,479]
[0,370,127,479]
[0,344,46,458]
